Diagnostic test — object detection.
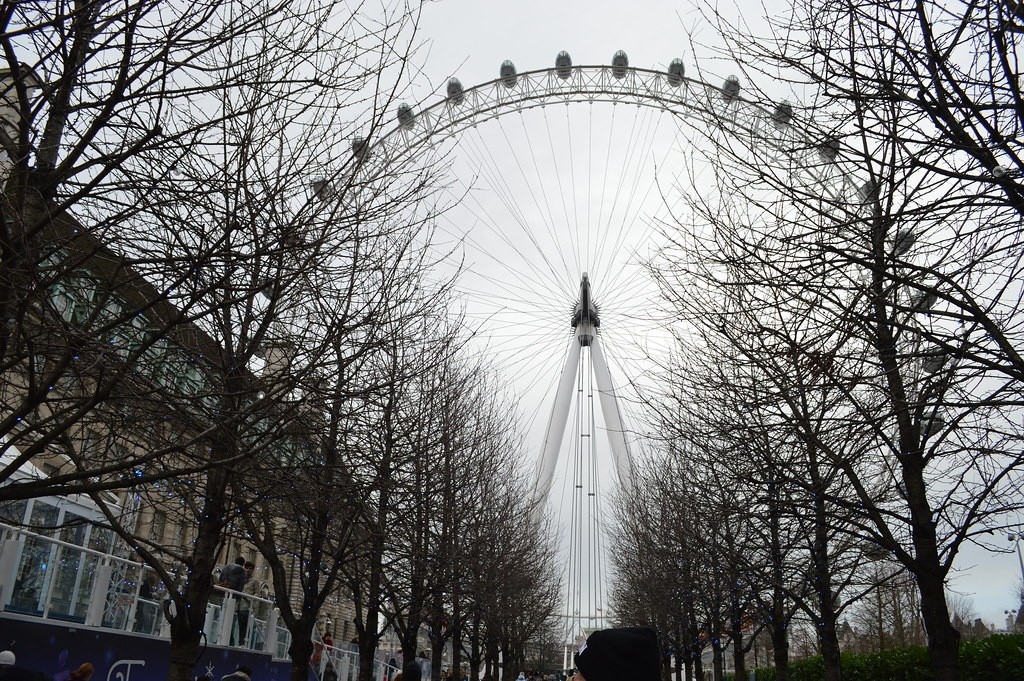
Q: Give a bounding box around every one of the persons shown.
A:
[229,561,263,647]
[312,633,428,681]
[220,666,253,681]
[134,578,160,633]
[218,556,246,646]
[59,662,95,681]
[442,668,578,681]
[0,650,23,681]
[574,627,663,681]
